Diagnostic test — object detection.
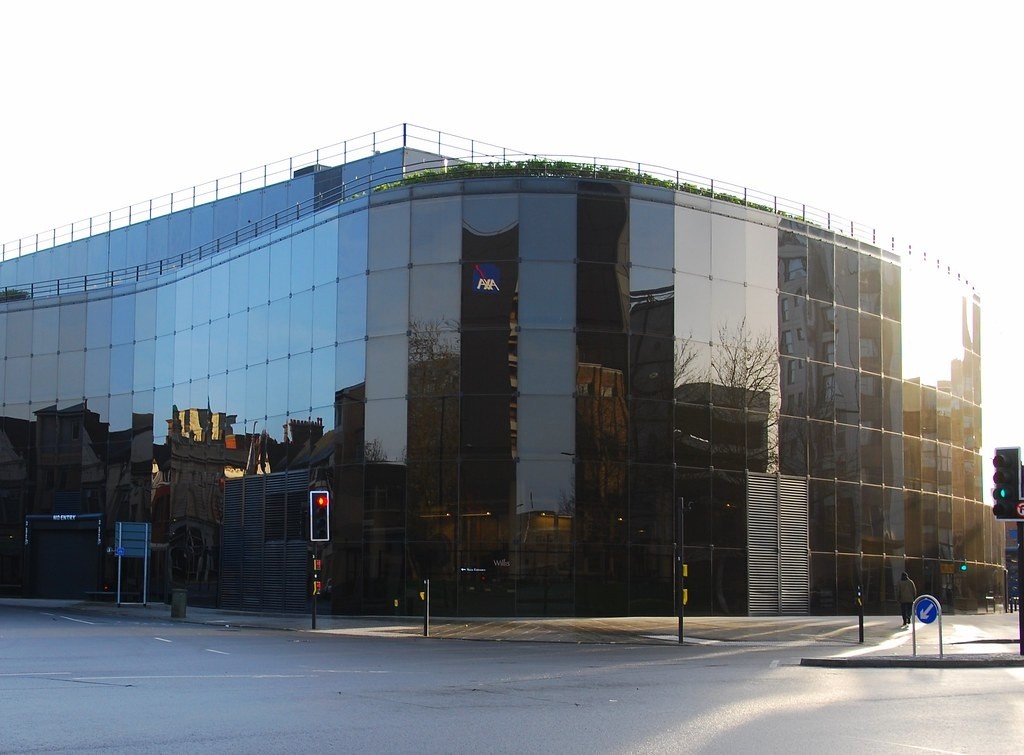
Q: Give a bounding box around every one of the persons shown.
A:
[897,571,916,625]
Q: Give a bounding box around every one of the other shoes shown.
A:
[903,623,906,625]
[908,618,910,624]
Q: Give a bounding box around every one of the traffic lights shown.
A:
[298,499,310,542]
[991,446,1024,521]
[310,489,331,544]
